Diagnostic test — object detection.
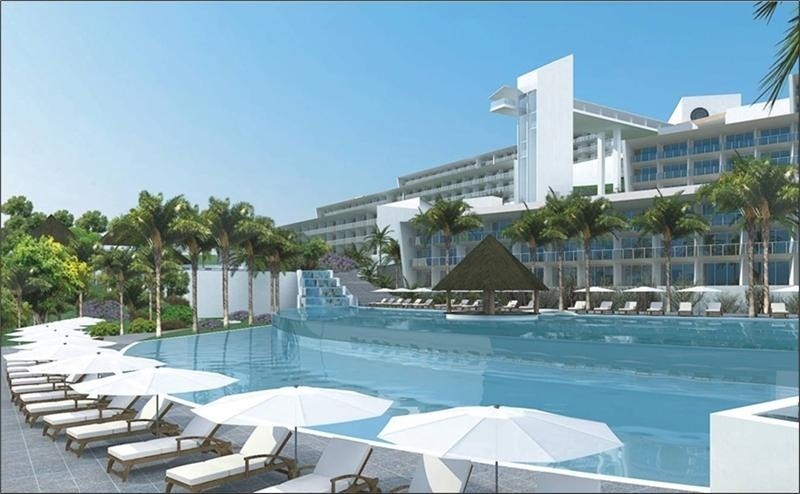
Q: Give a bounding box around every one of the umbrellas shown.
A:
[677,286,723,314]
[4,317,167,425]
[191,385,395,460]
[572,286,616,303]
[375,405,625,493]
[68,366,240,439]
[622,285,666,310]
[771,284,799,294]
[373,285,541,299]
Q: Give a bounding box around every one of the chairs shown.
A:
[369,297,534,312]
[647,301,665,315]
[618,301,640,315]
[705,302,723,317]
[568,300,587,315]
[66,396,179,458]
[7,361,111,427]
[677,302,693,316]
[163,426,297,494]
[42,396,140,442]
[388,455,473,492]
[769,303,789,318]
[106,417,232,481]
[592,301,614,316]
[256,437,381,493]
